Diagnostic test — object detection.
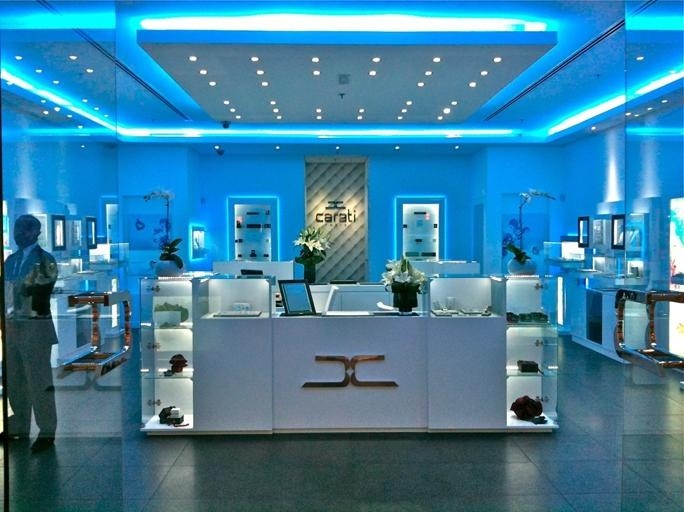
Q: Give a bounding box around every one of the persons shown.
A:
[3,215,59,452]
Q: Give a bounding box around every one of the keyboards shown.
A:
[212,309,261,318]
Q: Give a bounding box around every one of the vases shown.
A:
[392,283,420,312]
[303,263,315,283]
[155,260,184,276]
[507,259,536,274]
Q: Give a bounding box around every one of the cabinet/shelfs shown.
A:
[139,275,208,436]
[492,275,561,434]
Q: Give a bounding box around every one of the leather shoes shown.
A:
[0,431,30,439]
[30,437,55,450]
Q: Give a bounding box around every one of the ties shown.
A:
[15,250,23,278]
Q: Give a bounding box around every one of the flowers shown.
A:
[382,255,425,293]
[293,228,328,264]
[144,190,184,269]
[501,190,556,263]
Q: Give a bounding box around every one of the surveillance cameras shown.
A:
[221,121,230,128]
[216,148,224,155]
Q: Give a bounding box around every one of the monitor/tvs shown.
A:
[278,278,319,317]
